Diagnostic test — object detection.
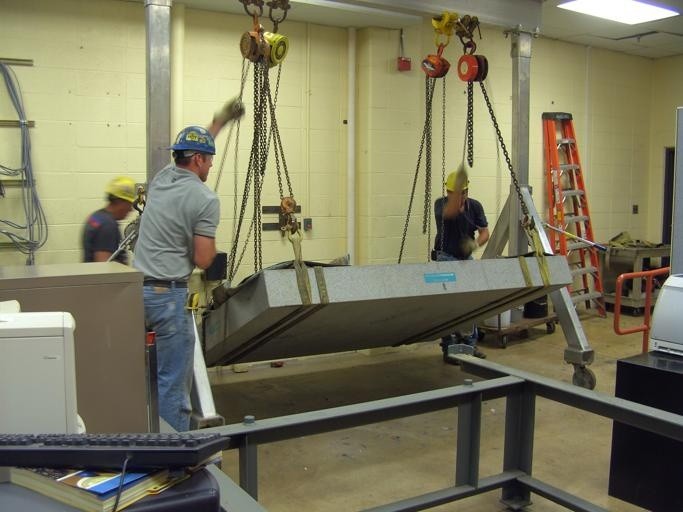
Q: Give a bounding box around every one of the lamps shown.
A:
[557,0,681,25]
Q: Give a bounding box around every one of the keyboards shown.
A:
[1,428,233,468]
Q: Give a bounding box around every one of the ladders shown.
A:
[542,111,607,318]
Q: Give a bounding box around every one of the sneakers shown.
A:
[472,341,488,359]
[440,343,461,366]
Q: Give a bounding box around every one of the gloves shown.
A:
[461,235,480,254]
[212,96,246,126]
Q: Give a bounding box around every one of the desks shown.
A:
[0,417,270,512]
[607,350,683,512]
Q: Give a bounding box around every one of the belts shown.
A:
[143,278,190,289]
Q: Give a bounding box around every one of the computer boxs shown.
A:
[0,309,80,433]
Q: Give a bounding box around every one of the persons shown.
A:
[434,168,488,364]
[78,176,137,265]
[130,96,248,432]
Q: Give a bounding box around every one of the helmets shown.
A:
[446,170,470,192]
[104,175,145,204]
[166,125,215,155]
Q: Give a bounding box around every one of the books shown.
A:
[8,448,226,511]
